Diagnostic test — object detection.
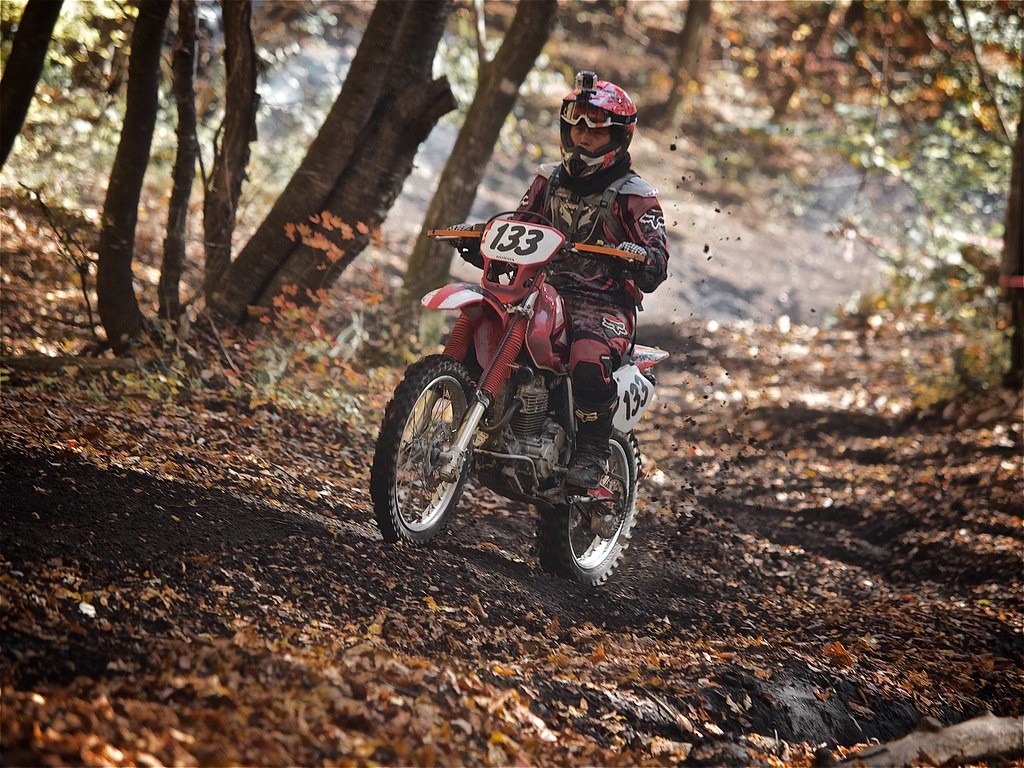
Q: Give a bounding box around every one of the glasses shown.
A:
[560,98,638,130]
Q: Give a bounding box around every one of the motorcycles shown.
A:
[367,210,672,589]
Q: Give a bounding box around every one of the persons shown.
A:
[435,79,670,490]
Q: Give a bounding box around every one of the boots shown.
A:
[565,390,620,490]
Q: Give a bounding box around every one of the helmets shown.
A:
[557,71,638,179]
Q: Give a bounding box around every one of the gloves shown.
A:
[446,223,472,248]
[612,241,648,272]
